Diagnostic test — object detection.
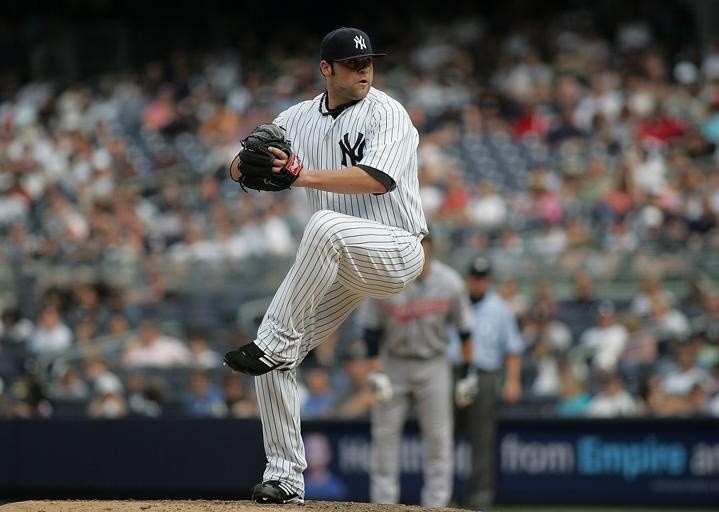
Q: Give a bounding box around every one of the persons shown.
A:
[1,7,719,416]
[354,224,479,508]
[447,256,528,508]
[222,23,431,505]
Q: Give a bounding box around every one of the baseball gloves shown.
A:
[237,123,303,190]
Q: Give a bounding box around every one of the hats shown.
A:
[320,27,387,62]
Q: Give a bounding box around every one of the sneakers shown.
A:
[253,480,303,504]
[224,342,296,375]
